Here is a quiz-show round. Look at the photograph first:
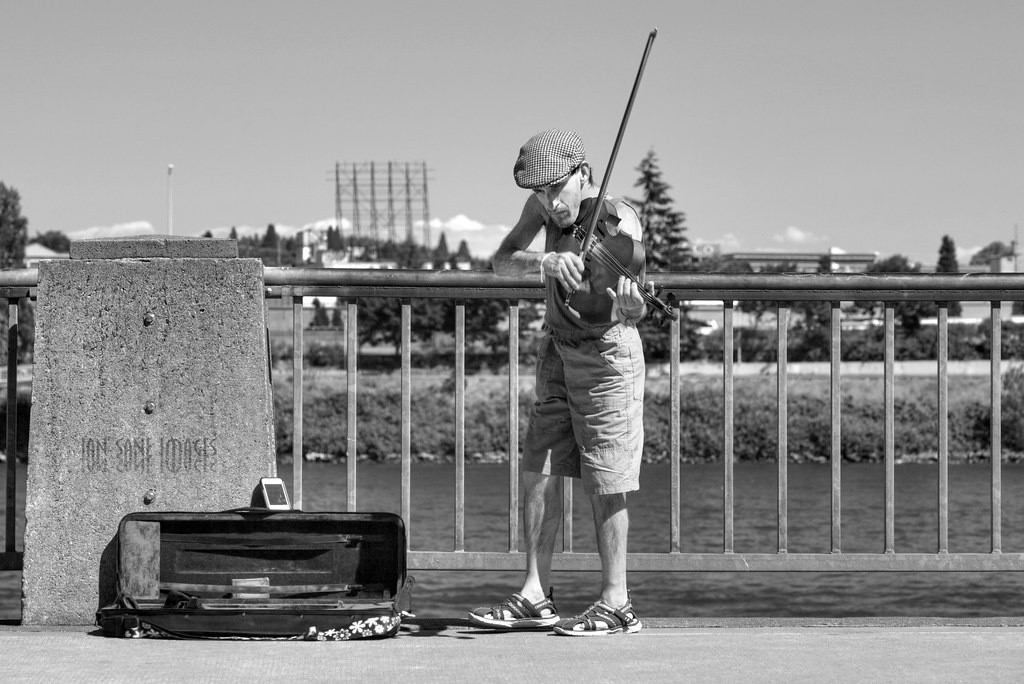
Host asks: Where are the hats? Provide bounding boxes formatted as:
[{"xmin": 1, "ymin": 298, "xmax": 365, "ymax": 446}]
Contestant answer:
[{"xmin": 513, "ymin": 129, "xmax": 585, "ymax": 187}]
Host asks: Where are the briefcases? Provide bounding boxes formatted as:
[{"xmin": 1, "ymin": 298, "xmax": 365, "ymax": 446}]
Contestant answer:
[{"xmin": 95, "ymin": 507, "xmax": 414, "ymax": 641}]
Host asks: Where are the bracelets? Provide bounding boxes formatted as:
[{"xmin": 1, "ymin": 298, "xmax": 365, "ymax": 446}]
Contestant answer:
[
  {"xmin": 620, "ymin": 308, "xmax": 642, "ymax": 329},
  {"xmin": 540, "ymin": 252, "xmax": 556, "ymax": 283}
]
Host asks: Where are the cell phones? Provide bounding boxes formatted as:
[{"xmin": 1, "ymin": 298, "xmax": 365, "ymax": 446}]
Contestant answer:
[{"xmin": 260, "ymin": 477, "xmax": 290, "ymax": 510}]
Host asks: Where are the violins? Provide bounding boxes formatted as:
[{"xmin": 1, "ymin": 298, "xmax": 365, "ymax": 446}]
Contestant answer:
[{"xmin": 554, "ymin": 195, "xmax": 679, "ymax": 328}]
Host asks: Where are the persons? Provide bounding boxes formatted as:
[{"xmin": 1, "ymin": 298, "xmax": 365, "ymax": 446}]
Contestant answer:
[{"xmin": 468, "ymin": 130, "xmax": 654, "ymax": 637}]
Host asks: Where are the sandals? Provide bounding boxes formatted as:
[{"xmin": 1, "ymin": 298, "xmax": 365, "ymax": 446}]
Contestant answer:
[
  {"xmin": 553, "ymin": 588, "xmax": 643, "ymax": 636},
  {"xmin": 467, "ymin": 586, "xmax": 560, "ymax": 628}
]
